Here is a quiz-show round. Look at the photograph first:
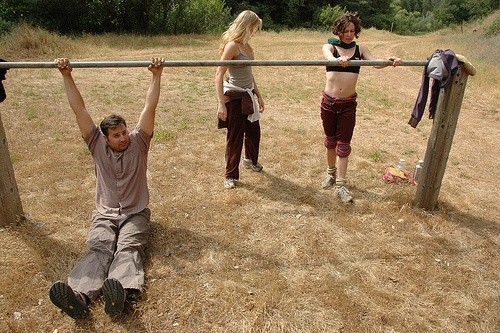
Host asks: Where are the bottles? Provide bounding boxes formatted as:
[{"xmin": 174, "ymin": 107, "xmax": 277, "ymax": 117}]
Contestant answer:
[
  {"xmin": 413, "ymin": 160, "xmax": 424, "ymax": 182},
  {"xmin": 396, "ymin": 159, "xmax": 405, "ymax": 172}
]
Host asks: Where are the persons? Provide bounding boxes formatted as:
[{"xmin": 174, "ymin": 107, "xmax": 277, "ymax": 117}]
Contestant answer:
[
  {"xmin": 216, "ymin": 10, "xmax": 265, "ymax": 189},
  {"xmin": 320, "ymin": 14, "xmax": 401, "ymax": 203},
  {"xmin": 49, "ymin": 57, "xmax": 165, "ymax": 320}
]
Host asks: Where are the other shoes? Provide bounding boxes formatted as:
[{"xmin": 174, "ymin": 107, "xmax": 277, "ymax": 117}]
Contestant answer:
[
  {"xmin": 223, "ymin": 178, "xmax": 235, "ymax": 189},
  {"xmin": 321, "ymin": 175, "xmax": 335, "ymax": 189},
  {"xmin": 332, "ymin": 186, "xmax": 353, "ymax": 202},
  {"xmin": 101, "ymin": 277, "xmax": 126, "ymax": 317},
  {"xmin": 49, "ymin": 281, "xmax": 89, "ymax": 319},
  {"xmin": 242, "ymin": 156, "xmax": 263, "ymax": 172}
]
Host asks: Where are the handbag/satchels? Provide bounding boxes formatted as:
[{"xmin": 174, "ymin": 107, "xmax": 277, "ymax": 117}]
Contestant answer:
[{"xmin": 383, "ymin": 165, "xmax": 418, "ymax": 186}]
